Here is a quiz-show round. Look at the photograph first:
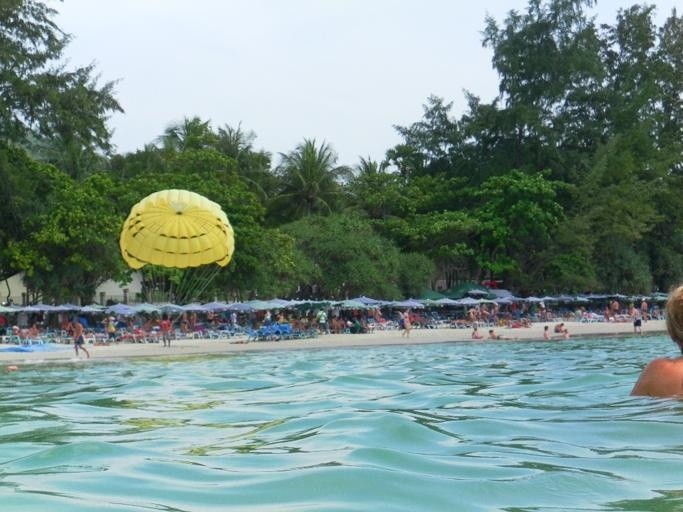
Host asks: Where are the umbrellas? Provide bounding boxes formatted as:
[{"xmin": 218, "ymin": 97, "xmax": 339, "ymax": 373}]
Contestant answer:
[{"xmin": 524, "ymin": 292, "xmax": 667, "ymax": 301}]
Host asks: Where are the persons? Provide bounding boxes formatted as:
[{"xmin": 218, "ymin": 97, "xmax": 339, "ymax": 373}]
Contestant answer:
[
  {"xmin": 1, "ymin": 297, "xmax": 663, "ymax": 338},
  {"xmin": 544, "ymin": 326, "xmax": 550, "ymax": 340},
  {"xmin": 65, "ymin": 318, "xmax": 90, "ymax": 359},
  {"xmin": 161, "ymin": 312, "xmax": 172, "ymax": 348},
  {"xmin": 628, "ymin": 286, "xmax": 683, "ymax": 400}
]
